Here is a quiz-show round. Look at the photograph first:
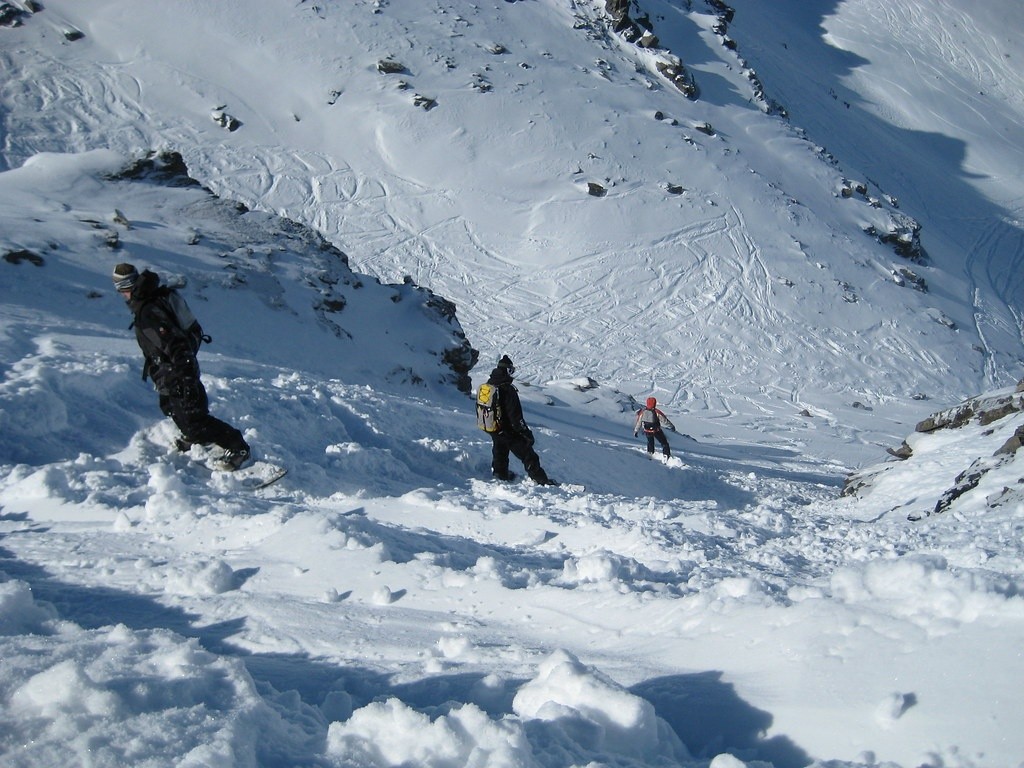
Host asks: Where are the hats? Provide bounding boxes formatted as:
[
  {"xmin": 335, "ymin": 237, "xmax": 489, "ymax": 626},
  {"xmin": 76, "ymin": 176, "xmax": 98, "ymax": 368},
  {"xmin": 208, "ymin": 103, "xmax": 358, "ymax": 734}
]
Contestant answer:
[
  {"xmin": 112, "ymin": 263, "xmax": 140, "ymax": 291},
  {"xmin": 498, "ymin": 355, "xmax": 515, "ymax": 370},
  {"xmin": 647, "ymin": 397, "xmax": 656, "ymax": 409}
]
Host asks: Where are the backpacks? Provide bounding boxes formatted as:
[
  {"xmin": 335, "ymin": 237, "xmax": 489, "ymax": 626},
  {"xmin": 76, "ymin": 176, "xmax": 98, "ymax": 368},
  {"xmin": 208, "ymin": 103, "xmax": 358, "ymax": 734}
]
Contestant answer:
[
  {"xmin": 475, "ymin": 381, "xmax": 511, "ymax": 434},
  {"xmin": 641, "ymin": 407, "xmax": 660, "ymax": 430},
  {"xmin": 134, "ymin": 284, "xmax": 212, "ymax": 360}
]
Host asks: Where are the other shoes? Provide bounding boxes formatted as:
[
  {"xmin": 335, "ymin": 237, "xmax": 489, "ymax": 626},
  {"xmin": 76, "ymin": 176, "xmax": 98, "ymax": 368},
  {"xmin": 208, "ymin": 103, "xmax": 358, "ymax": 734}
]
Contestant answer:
[
  {"xmin": 173, "ymin": 434, "xmax": 214, "ymax": 452},
  {"xmin": 493, "ymin": 470, "xmax": 515, "ymax": 481},
  {"xmin": 537, "ymin": 479, "xmax": 558, "ymax": 487},
  {"xmin": 214, "ymin": 446, "xmax": 251, "ymax": 472}
]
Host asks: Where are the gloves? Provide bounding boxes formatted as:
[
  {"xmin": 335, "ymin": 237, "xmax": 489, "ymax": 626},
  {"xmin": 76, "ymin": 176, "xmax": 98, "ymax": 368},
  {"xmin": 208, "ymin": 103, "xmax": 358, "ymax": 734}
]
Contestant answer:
[
  {"xmin": 634, "ymin": 431, "xmax": 638, "ymax": 438},
  {"xmin": 520, "ymin": 426, "xmax": 535, "ymax": 446},
  {"xmin": 669, "ymin": 427, "xmax": 676, "ymax": 433}
]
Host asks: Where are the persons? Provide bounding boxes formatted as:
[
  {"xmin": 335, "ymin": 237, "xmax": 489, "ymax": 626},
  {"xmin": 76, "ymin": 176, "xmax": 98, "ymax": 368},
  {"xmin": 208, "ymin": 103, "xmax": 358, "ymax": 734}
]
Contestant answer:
[
  {"xmin": 634, "ymin": 397, "xmax": 675, "ymax": 459},
  {"xmin": 113, "ymin": 263, "xmax": 251, "ymax": 472},
  {"xmin": 486, "ymin": 355, "xmax": 557, "ymax": 486}
]
